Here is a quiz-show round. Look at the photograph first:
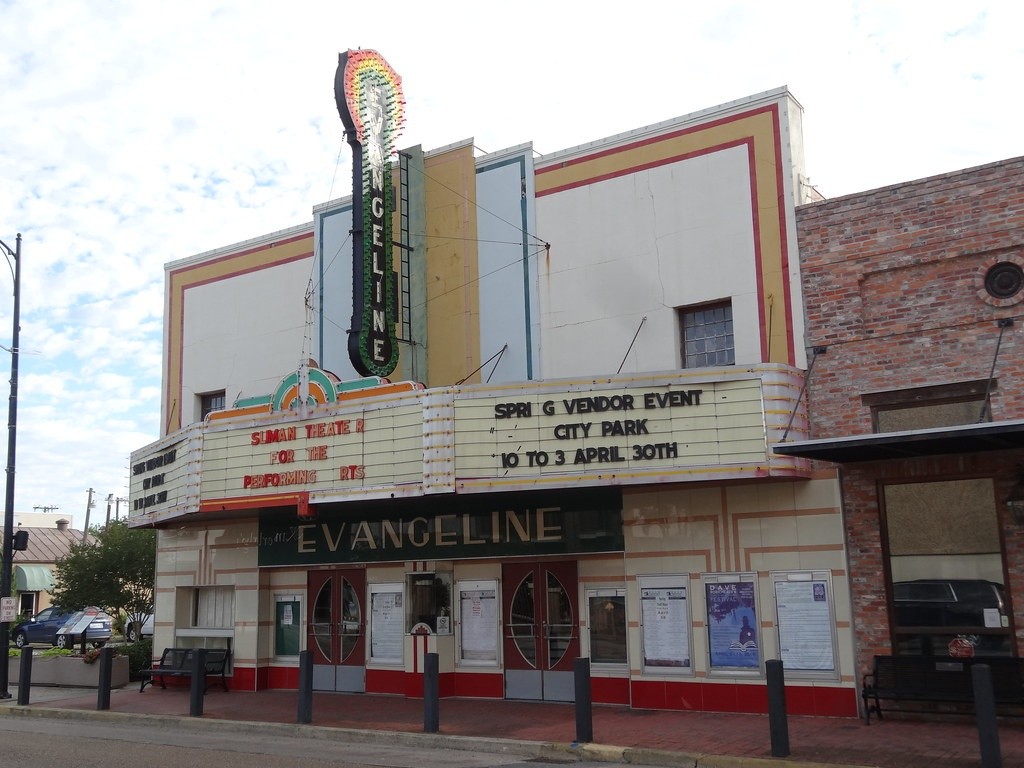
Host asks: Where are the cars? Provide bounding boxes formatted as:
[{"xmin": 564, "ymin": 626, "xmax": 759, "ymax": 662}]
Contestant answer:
[
  {"xmin": 892, "ymin": 577, "xmax": 1005, "ymax": 629},
  {"xmin": 11, "ymin": 606, "xmax": 113, "ymax": 650},
  {"xmin": 125, "ymin": 602, "xmax": 154, "ymax": 644}
]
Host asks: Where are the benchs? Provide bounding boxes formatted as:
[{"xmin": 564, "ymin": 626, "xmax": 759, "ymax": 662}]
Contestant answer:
[
  {"xmin": 139, "ymin": 648, "xmax": 231, "ymax": 695},
  {"xmin": 862, "ymin": 655, "xmax": 1024, "ymax": 725}
]
